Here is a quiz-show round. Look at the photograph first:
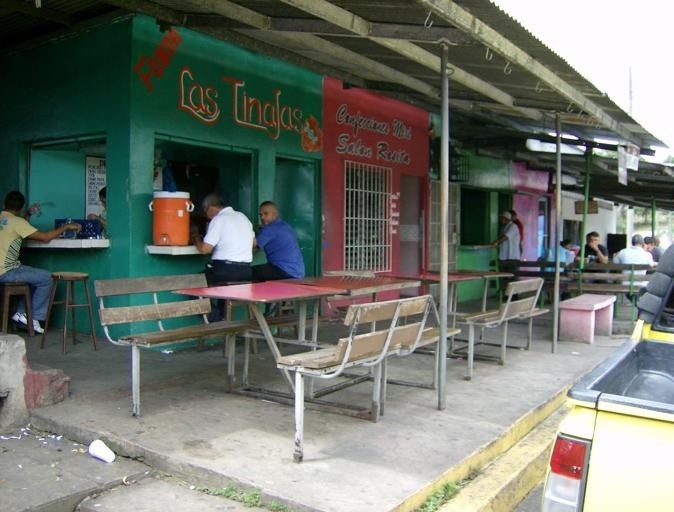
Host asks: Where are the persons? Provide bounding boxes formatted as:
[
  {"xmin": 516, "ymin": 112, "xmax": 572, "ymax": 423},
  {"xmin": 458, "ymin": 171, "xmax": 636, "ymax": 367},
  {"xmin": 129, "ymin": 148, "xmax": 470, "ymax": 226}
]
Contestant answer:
[
  {"xmin": 551, "ymin": 233, "xmax": 663, "ymax": 302},
  {"xmin": 491, "ymin": 211, "xmax": 520, "ymax": 302},
  {"xmin": 0, "ymin": 191, "xmax": 82, "ymax": 335},
  {"xmin": 86, "ymin": 185, "xmax": 106, "ymax": 236},
  {"xmin": 256, "ymin": 201, "xmax": 305, "ymax": 318},
  {"xmin": 510, "ymin": 210, "xmax": 523, "ymax": 257},
  {"xmin": 189, "ymin": 192, "xmax": 258, "ymax": 324}
]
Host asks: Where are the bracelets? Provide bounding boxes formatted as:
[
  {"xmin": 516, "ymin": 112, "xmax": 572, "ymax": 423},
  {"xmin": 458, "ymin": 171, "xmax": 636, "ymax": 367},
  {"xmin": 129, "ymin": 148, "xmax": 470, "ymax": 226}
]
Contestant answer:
[
  {"xmin": 27, "ymin": 211, "xmax": 32, "ymax": 216},
  {"xmin": 493, "ymin": 241, "xmax": 498, "ymax": 246}
]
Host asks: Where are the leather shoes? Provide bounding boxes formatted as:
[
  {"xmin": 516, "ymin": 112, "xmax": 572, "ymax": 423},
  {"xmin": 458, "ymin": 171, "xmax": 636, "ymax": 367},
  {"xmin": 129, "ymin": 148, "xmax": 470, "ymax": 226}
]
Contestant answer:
[{"xmin": 263, "ymin": 303, "xmax": 276, "ymax": 318}]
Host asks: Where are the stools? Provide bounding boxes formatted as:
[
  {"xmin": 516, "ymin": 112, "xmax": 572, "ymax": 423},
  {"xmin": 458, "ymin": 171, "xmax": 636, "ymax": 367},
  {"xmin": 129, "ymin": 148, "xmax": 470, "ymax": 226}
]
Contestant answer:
[
  {"xmin": 42, "ymin": 272, "xmax": 99, "ymax": 355},
  {"xmin": 0, "ymin": 280, "xmax": 35, "ymax": 336}
]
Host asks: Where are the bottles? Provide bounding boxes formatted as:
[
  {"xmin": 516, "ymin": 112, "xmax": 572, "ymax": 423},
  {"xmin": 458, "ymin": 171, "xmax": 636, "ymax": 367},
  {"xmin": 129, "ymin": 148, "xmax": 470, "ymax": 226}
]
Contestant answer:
[{"xmin": 63, "ymin": 217, "xmax": 75, "ymax": 239}]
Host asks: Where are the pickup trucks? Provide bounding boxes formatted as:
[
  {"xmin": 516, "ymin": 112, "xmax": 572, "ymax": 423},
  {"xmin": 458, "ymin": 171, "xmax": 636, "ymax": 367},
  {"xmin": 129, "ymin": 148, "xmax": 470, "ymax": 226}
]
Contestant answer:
[{"xmin": 541, "ymin": 243, "xmax": 673, "ymax": 512}]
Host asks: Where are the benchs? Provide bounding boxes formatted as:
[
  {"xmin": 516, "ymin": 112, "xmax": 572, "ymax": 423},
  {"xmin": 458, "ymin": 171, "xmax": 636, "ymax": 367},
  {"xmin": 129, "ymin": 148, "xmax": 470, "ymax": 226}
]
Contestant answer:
[
  {"xmin": 489, "ymin": 258, "xmax": 563, "ymax": 319},
  {"xmin": 565, "ymin": 262, "xmax": 651, "ymax": 325},
  {"xmin": 557, "ymin": 292, "xmax": 617, "ymax": 344}
]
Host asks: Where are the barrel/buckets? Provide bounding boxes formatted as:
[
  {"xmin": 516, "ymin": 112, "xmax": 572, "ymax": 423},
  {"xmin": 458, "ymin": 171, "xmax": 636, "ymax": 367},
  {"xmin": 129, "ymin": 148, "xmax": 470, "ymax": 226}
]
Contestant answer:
[{"xmin": 148, "ymin": 189, "xmax": 194, "ymax": 246}]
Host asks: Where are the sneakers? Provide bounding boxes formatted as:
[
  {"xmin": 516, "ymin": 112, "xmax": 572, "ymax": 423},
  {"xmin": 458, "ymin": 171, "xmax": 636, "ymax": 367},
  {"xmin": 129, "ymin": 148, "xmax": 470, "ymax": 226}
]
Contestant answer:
[{"xmin": 12, "ymin": 312, "xmax": 44, "ymax": 334}]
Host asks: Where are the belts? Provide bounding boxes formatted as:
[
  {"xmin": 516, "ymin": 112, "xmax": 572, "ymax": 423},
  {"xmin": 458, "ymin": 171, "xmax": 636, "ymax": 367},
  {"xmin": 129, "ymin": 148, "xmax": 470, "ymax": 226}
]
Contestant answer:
[{"xmin": 224, "ymin": 261, "xmax": 249, "ymax": 266}]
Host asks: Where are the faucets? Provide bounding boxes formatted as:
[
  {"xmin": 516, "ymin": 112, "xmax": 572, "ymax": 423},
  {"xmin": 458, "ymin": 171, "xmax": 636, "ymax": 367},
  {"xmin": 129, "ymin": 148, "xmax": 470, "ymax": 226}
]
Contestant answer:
[{"xmin": 160, "ymin": 232, "xmax": 169, "ymax": 242}]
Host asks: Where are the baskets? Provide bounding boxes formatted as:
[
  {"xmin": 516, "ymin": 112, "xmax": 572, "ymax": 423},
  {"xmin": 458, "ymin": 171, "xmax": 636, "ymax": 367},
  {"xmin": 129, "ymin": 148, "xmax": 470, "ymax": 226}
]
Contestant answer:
[{"xmin": 55, "ymin": 219, "xmax": 101, "ymax": 239}]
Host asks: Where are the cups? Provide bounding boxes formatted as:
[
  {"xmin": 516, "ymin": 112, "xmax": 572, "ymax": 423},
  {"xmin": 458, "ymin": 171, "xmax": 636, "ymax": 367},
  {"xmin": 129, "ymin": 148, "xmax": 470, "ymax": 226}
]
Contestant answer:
[{"xmin": 30, "ymin": 205, "xmax": 41, "ymax": 218}]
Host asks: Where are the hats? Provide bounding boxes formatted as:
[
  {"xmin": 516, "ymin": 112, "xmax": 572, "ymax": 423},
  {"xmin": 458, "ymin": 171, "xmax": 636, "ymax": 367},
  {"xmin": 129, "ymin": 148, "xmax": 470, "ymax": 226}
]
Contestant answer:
[{"xmin": 499, "ymin": 211, "xmax": 511, "ymax": 219}]
